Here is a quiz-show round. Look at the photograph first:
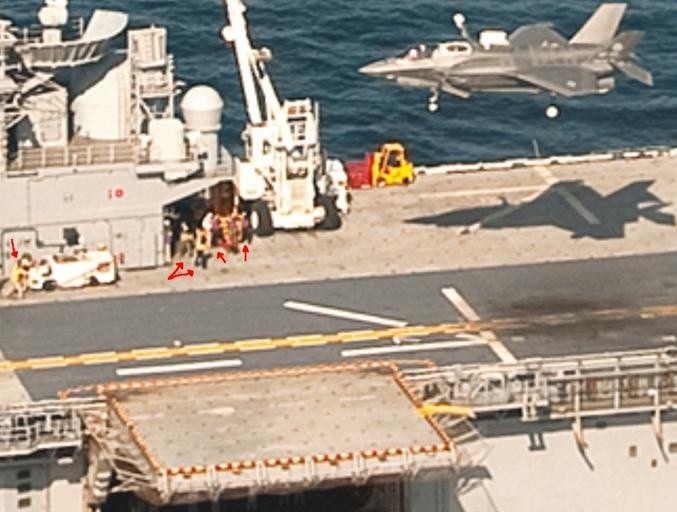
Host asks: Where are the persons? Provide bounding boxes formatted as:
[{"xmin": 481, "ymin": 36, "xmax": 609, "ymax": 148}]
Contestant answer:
[
  {"xmin": 174, "ymin": 207, "xmax": 254, "ymax": 269},
  {"xmin": 7, "ymin": 258, "xmax": 31, "ymax": 299}
]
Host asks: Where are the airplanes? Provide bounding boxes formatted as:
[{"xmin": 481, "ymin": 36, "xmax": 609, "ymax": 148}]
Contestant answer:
[{"xmin": 352, "ymin": 1, "xmax": 657, "ymax": 121}]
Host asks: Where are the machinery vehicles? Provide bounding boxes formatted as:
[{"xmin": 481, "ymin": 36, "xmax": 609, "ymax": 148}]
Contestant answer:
[
  {"xmin": 27, "ymin": 248, "xmax": 119, "ymax": 294},
  {"xmin": 215, "ymin": 1, "xmax": 349, "ymax": 239}
]
